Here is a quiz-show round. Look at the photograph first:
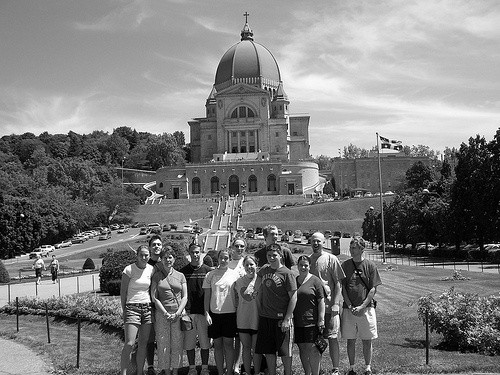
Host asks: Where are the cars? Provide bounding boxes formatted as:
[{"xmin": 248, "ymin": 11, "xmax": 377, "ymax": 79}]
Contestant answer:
[
  {"xmin": 259, "ymin": 191, "xmax": 394, "ymax": 212},
  {"xmin": 181, "ymin": 225, "xmax": 203, "ymax": 233},
  {"xmin": 385, "ymin": 242, "xmax": 500, "ymax": 253},
  {"xmin": 29, "ymin": 223, "xmax": 129, "ymax": 260},
  {"xmin": 139, "ymin": 223, "xmax": 178, "ymax": 236},
  {"xmin": 236, "ymin": 225, "xmax": 350, "ymax": 243},
  {"xmin": 131, "ymin": 221, "xmax": 146, "ymax": 229},
  {"xmin": 423, "ymin": 189, "xmax": 429, "ymax": 194}
]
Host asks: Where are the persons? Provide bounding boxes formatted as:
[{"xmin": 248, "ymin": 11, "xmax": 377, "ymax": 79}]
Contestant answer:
[
  {"xmin": 294, "ymin": 256, "xmax": 326, "ymax": 375},
  {"xmin": 340, "ymin": 236, "xmax": 383, "ymax": 375},
  {"xmin": 178, "ymin": 243, "xmax": 215, "ymax": 375},
  {"xmin": 46, "ymin": 255, "xmax": 59, "ymax": 282},
  {"xmin": 254, "ymin": 224, "xmax": 296, "ymax": 375},
  {"xmin": 307, "ymin": 231, "xmax": 346, "ymax": 375},
  {"xmin": 32, "ymin": 252, "xmax": 47, "ymax": 283},
  {"xmin": 130, "ymin": 236, "xmax": 162, "ymax": 375},
  {"xmin": 222, "ymin": 238, "xmax": 251, "ymax": 375},
  {"xmin": 234, "ymin": 255, "xmax": 263, "ymax": 375},
  {"xmin": 254, "ymin": 244, "xmax": 297, "ymax": 374},
  {"xmin": 120, "ymin": 245, "xmax": 155, "ymax": 375},
  {"xmin": 151, "ymin": 246, "xmax": 188, "ymax": 375},
  {"xmin": 202, "ymin": 248, "xmax": 241, "ymax": 375}
]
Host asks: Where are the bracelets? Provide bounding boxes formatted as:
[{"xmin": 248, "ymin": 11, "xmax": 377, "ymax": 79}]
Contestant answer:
[
  {"xmin": 175, "ymin": 313, "xmax": 178, "ymax": 320},
  {"xmin": 204, "ymin": 312, "xmax": 209, "ymax": 316}
]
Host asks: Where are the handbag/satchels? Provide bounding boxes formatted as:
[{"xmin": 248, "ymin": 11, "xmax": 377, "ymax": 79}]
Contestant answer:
[
  {"xmin": 180, "ymin": 313, "xmax": 193, "ymax": 331},
  {"xmin": 314, "ymin": 326, "xmax": 328, "ymax": 355}
]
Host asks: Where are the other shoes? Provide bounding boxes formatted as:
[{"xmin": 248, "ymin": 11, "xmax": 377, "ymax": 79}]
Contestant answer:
[
  {"xmin": 188, "ymin": 368, "xmax": 197, "ymax": 375},
  {"xmin": 319, "ymin": 370, "xmax": 325, "ymax": 375},
  {"xmin": 331, "ymin": 369, "xmax": 339, "ymax": 375},
  {"xmin": 200, "ymin": 368, "xmax": 210, "ymax": 375},
  {"xmin": 148, "ymin": 368, "xmax": 156, "ymax": 375},
  {"xmin": 348, "ymin": 368, "xmax": 357, "ymax": 375},
  {"xmin": 364, "ymin": 370, "xmax": 373, "ymax": 375}
]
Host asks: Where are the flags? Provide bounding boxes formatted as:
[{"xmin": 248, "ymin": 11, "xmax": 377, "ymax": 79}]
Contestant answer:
[{"xmin": 377, "ymin": 135, "xmax": 404, "ymax": 155}]
